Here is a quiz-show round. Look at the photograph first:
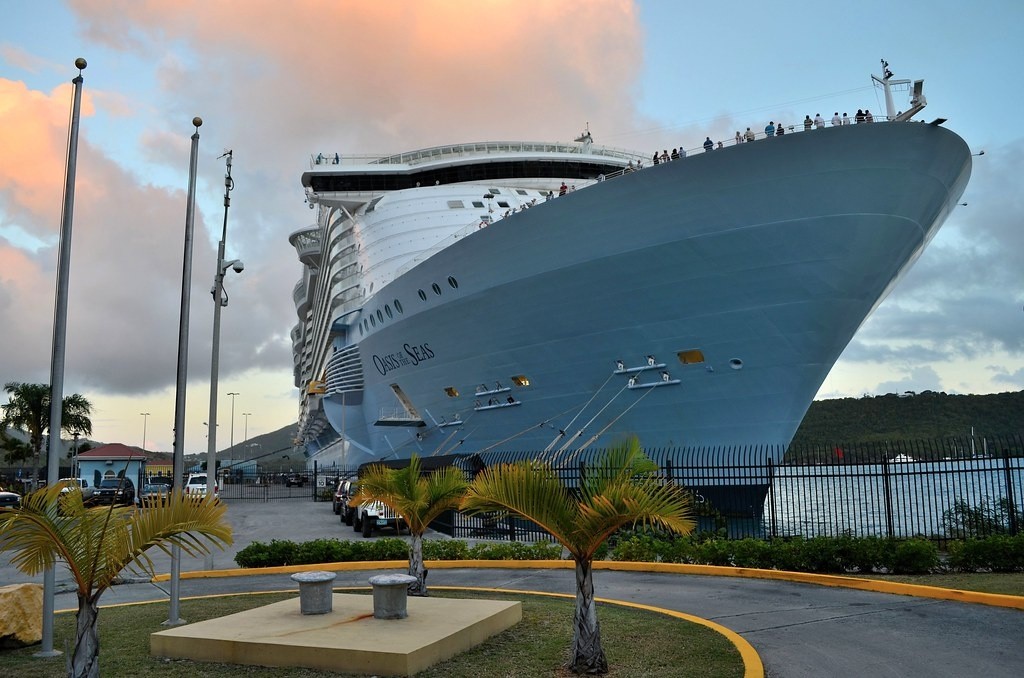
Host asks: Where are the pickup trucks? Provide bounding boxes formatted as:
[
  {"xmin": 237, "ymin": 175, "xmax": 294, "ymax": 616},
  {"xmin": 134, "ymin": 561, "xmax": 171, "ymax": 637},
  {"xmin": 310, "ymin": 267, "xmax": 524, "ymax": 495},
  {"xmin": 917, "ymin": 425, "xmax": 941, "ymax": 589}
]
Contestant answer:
[{"xmin": 58, "ymin": 478, "xmax": 96, "ymax": 501}]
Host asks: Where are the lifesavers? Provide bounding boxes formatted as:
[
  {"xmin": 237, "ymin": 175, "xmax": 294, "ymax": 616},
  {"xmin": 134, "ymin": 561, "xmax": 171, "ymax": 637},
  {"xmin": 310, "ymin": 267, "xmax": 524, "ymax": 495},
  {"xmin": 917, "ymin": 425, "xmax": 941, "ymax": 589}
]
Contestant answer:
[{"xmin": 479, "ymin": 222, "xmax": 488, "ymax": 229}]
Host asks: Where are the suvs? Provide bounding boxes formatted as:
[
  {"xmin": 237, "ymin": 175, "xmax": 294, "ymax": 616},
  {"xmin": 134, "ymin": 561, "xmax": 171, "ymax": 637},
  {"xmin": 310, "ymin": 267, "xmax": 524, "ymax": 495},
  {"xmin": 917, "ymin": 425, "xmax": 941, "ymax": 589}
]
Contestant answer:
[
  {"xmin": 183, "ymin": 472, "xmax": 219, "ymax": 505},
  {"xmin": 92, "ymin": 477, "xmax": 135, "ymax": 504}
]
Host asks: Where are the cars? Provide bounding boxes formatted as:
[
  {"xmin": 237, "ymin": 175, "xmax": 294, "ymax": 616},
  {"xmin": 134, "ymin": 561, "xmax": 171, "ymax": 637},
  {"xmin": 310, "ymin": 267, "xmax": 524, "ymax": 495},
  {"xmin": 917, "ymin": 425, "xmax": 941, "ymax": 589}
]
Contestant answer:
[
  {"xmin": 332, "ymin": 479, "xmax": 423, "ymax": 538},
  {"xmin": 140, "ymin": 482, "xmax": 171, "ymax": 506},
  {"xmin": 288, "ymin": 473, "xmax": 303, "ymax": 486},
  {"xmin": 0, "ymin": 491, "xmax": 23, "ymax": 514}
]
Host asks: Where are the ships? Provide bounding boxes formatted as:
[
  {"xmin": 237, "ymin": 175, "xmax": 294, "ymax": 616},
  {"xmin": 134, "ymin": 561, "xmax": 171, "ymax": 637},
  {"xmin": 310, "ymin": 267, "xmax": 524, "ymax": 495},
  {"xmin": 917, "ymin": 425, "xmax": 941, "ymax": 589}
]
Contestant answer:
[{"xmin": 289, "ymin": 56, "xmax": 973, "ymax": 541}]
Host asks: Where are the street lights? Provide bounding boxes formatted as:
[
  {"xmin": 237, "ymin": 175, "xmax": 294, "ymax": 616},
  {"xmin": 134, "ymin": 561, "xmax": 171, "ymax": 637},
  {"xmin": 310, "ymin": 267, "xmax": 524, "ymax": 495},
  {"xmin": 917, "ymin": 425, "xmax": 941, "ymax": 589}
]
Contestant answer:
[
  {"xmin": 139, "ymin": 412, "xmax": 150, "ymax": 453},
  {"xmin": 228, "ymin": 392, "xmax": 241, "ymax": 467},
  {"xmin": 242, "ymin": 412, "xmax": 251, "ymax": 443}
]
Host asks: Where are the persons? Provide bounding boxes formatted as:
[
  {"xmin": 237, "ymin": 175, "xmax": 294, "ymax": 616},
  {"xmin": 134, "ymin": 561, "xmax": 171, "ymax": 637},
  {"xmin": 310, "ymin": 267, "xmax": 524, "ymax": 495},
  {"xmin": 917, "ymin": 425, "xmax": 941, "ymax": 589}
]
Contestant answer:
[
  {"xmin": 897, "ymin": 111, "xmax": 902, "ymax": 116},
  {"xmin": 765, "ymin": 121, "xmax": 775, "ymax": 137},
  {"xmin": 832, "ymin": 112, "xmax": 850, "ymax": 126},
  {"xmin": 16, "ymin": 469, "xmax": 23, "ymax": 479},
  {"xmin": 505, "ymin": 199, "xmax": 537, "ymax": 217},
  {"xmin": 804, "ymin": 115, "xmax": 813, "ymax": 130},
  {"xmin": 703, "ymin": 137, "xmax": 723, "ymax": 151},
  {"xmin": 334, "ymin": 153, "xmax": 340, "ymax": 164},
  {"xmin": 627, "ymin": 160, "xmax": 643, "ymax": 172},
  {"xmin": 736, "ymin": 127, "xmax": 755, "ymax": 144},
  {"xmin": 855, "ymin": 109, "xmax": 872, "ymax": 123},
  {"xmin": 814, "ymin": 114, "xmax": 825, "ymax": 129},
  {"xmin": 546, "ymin": 191, "xmax": 554, "ymax": 199},
  {"xmin": 653, "ymin": 147, "xmax": 686, "ymax": 166},
  {"xmin": 559, "ymin": 182, "xmax": 567, "ymax": 196},
  {"xmin": 316, "ymin": 153, "xmax": 325, "ymax": 164},
  {"xmin": 776, "ymin": 124, "xmax": 784, "ymax": 136},
  {"xmin": 885, "ymin": 69, "xmax": 891, "ymax": 76}
]
